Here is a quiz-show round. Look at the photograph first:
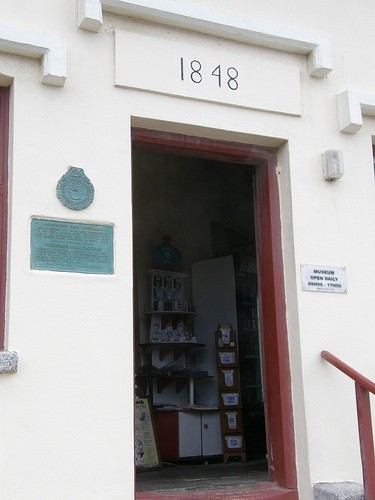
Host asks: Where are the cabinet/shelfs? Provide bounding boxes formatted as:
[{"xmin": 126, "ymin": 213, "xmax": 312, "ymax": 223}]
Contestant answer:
[
  {"xmin": 233, "ymin": 269, "xmax": 264, "ymax": 428},
  {"xmin": 132, "ymin": 230, "xmax": 245, "ymax": 464}
]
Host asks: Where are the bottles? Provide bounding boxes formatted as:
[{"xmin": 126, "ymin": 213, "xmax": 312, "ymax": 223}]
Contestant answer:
[
  {"xmin": 164, "ymin": 272, "xmax": 172, "ymax": 297},
  {"xmin": 153, "ymin": 271, "xmax": 163, "ymax": 299},
  {"xmin": 174, "ymin": 274, "xmax": 181, "ymax": 290}
]
